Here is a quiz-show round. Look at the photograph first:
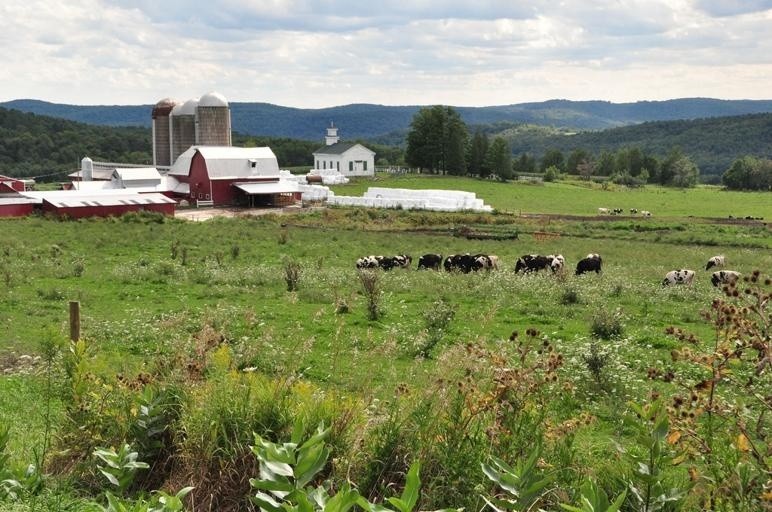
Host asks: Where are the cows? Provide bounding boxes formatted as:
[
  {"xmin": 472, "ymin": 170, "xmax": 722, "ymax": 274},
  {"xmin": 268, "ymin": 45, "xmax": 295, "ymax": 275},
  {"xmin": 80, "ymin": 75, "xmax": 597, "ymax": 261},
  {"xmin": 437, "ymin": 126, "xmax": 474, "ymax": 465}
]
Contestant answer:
[
  {"xmin": 661, "ymin": 269, "xmax": 696, "ymax": 288},
  {"xmin": 702, "ymin": 256, "xmax": 727, "ymax": 271},
  {"xmin": 598, "ymin": 207, "xmax": 653, "ymax": 217},
  {"xmin": 587, "ymin": 253, "xmax": 601, "ymax": 262},
  {"xmin": 711, "ymin": 270, "xmax": 742, "ymax": 289},
  {"xmin": 575, "ymin": 259, "xmax": 600, "ymax": 276},
  {"xmin": 514, "ymin": 253, "xmax": 565, "ymax": 276},
  {"xmin": 355, "ymin": 252, "xmax": 500, "ymax": 275}
]
